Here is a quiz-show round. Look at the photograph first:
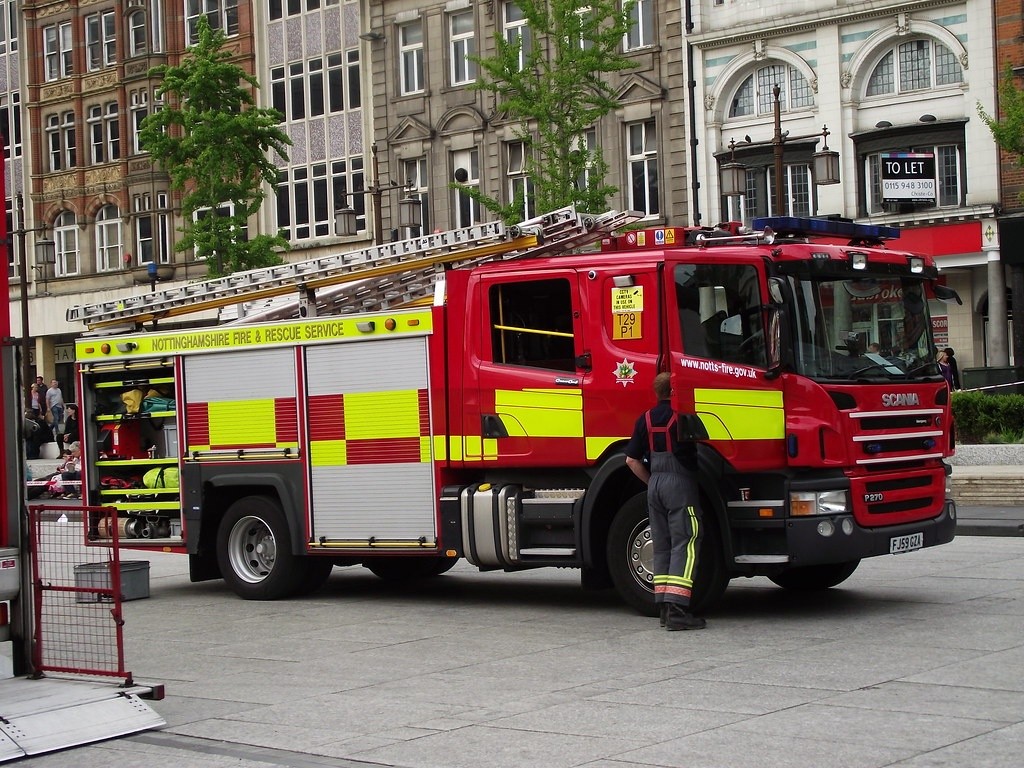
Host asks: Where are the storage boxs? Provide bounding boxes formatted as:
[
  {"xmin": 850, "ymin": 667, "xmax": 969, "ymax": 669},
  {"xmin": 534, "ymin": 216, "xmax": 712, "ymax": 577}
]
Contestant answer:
[{"xmin": 74, "ymin": 561, "xmax": 150, "ymax": 603}]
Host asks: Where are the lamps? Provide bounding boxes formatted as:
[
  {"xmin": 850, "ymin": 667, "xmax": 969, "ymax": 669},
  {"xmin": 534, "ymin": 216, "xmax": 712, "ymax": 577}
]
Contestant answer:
[{"xmin": 359, "ymin": 33, "xmax": 384, "ymax": 42}]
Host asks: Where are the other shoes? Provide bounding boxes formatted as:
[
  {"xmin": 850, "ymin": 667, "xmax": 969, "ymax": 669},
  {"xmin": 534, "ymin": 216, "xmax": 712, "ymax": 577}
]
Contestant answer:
[
  {"xmin": 660, "ymin": 605, "xmax": 666, "ymax": 627},
  {"xmin": 77, "ymin": 494, "xmax": 83, "ymax": 500},
  {"xmin": 63, "ymin": 492, "xmax": 78, "ymax": 500},
  {"xmin": 665, "ymin": 602, "xmax": 707, "ymax": 630}
]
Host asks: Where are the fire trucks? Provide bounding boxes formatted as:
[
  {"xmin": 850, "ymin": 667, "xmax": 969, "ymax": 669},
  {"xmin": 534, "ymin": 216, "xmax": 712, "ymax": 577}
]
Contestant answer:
[{"xmin": 65, "ymin": 199, "xmax": 959, "ymax": 610}]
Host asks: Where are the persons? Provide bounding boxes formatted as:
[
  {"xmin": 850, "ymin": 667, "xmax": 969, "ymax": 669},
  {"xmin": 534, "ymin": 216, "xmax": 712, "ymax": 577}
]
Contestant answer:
[
  {"xmin": 30, "ymin": 376, "xmax": 48, "ymax": 422},
  {"xmin": 937, "ymin": 348, "xmax": 961, "ymax": 392},
  {"xmin": 46, "ymin": 379, "xmax": 65, "ymax": 435},
  {"xmin": 625, "ymin": 373, "xmax": 709, "ymax": 631},
  {"xmin": 56, "ymin": 404, "xmax": 79, "ymax": 459},
  {"xmin": 27, "ymin": 441, "xmax": 83, "ymax": 500},
  {"xmin": 25, "ymin": 408, "xmax": 55, "ymax": 459},
  {"xmin": 868, "ymin": 343, "xmax": 880, "ymax": 353},
  {"xmin": 893, "ymin": 345, "xmax": 904, "ymax": 356}
]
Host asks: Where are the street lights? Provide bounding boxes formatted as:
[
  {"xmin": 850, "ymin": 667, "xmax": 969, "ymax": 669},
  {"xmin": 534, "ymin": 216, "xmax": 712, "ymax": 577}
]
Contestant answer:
[
  {"xmin": 328, "ymin": 139, "xmax": 424, "ymax": 245},
  {"xmin": 712, "ymin": 83, "xmax": 844, "ymax": 224},
  {"xmin": 6, "ymin": 192, "xmax": 58, "ymax": 454}
]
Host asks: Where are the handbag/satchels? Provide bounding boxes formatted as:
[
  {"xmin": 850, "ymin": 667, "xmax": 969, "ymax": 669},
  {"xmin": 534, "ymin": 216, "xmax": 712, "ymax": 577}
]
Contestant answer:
[{"xmin": 44, "ymin": 410, "xmax": 55, "ymax": 424}]
